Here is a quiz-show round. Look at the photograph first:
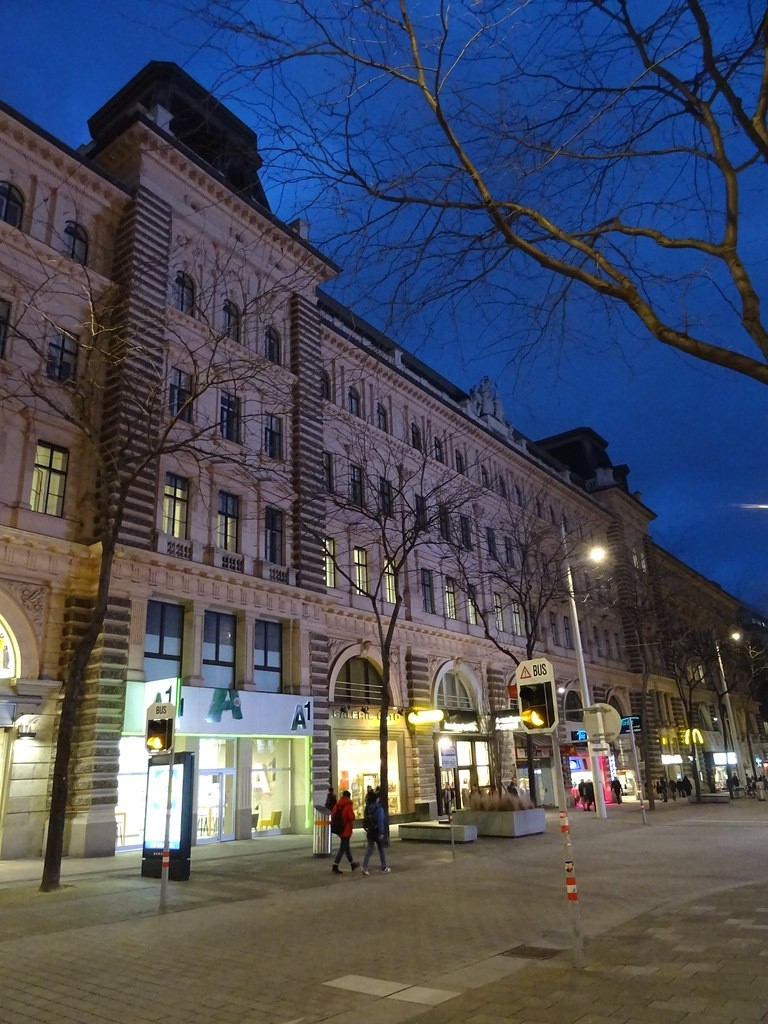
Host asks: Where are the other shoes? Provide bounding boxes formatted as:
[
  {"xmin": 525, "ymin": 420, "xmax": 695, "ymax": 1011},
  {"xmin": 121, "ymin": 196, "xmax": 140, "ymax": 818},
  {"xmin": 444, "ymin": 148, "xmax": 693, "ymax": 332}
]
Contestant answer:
[
  {"xmin": 363, "ymin": 870, "xmax": 369, "ymax": 875},
  {"xmin": 351, "ymin": 862, "xmax": 359, "ymax": 871},
  {"xmin": 588, "ymin": 809, "xmax": 591, "ymax": 811},
  {"xmin": 584, "ymin": 809, "xmax": 586, "ymax": 811},
  {"xmin": 382, "ymin": 866, "xmax": 391, "ymax": 873},
  {"xmin": 663, "ymin": 800, "xmax": 667, "ymax": 802},
  {"xmin": 332, "ymin": 864, "xmax": 343, "ymax": 874}
]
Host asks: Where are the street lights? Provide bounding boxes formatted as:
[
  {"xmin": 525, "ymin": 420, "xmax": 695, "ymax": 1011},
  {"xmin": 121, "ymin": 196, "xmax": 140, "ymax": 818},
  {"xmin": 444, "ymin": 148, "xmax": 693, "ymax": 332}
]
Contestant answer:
[
  {"xmin": 711, "ymin": 626, "xmax": 749, "ymax": 800},
  {"xmin": 560, "ymin": 521, "xmax": 609, "ymax": 823}
]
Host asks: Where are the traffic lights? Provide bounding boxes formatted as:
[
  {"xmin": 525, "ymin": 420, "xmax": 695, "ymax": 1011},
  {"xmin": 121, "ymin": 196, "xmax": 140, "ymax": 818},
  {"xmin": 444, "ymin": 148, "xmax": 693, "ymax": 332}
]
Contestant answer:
[
  {"xmin": 148, "ymin": 719, "xmax": 166, "ymax": 750},
  {"xmin": 513, "ymin": 657, "xmax": 560, "ymax": 736}
]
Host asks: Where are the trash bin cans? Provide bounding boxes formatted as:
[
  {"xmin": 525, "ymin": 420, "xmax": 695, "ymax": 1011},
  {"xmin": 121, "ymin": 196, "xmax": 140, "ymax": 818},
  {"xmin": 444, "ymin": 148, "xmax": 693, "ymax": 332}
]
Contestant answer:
[
  {"xmin": 313, "ymin": 804, "xmax": 331, "ymax": 856},
  {"xmin": 756, "ymin": 782, "xmax": 766, "ymax": 800}
]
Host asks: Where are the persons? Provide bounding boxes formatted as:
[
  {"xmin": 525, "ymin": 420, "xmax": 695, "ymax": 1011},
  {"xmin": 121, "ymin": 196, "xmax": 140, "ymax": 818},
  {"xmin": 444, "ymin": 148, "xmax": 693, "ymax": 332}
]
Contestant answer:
[
  {"xmin": 375, "ymin": 786, "xmax": 382, "ymax": 807},
  {"xmin": 586, "ymin": 779, "xmax": 596, "ymax": 812},
  {"xmin": 611, "ymin": 777, "xmax": 622, "ymax": 804},
  {"xmin": 732, "ymin": 772, "xmax": 768, "ymax": 795},
  {"xmin": 332, "ymin": 790, "xmax": 360, "ymax": 874},
  {"xmin": 325, "ymin": 787, "xmax": 337, "ymax": 811},
  {"xmin": 578, "ymin": 779, "xmax": 589, "ymax": 811},
  {"xmin": 360, "ymin": 793, "xmax": 391, "ymax": 875},
  {"xmin": 441, "ymin": 782, "xmax": 454, "ymax": 815},
  {"xmin": 645, "ymin": 775, "xmax": 693, "ymax": 802},
  {"xmin": 365, "ymin": 785, "xmax": 376, "ymax": 828}
]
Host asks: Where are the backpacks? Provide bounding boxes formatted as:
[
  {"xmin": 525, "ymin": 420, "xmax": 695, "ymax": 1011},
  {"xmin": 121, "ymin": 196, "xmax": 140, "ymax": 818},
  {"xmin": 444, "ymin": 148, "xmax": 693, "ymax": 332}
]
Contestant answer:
[
  {"xmin": 331, "ymin": 804, "xmax": 344, "ymax": 835},
  {"xmin": 362, "ymin": 806, "xmax": 381, "ymax": 833}
]
